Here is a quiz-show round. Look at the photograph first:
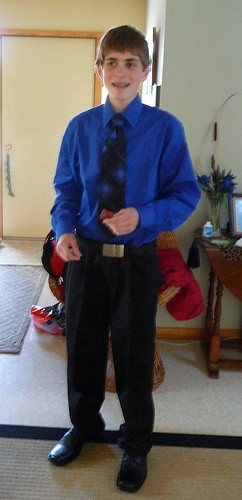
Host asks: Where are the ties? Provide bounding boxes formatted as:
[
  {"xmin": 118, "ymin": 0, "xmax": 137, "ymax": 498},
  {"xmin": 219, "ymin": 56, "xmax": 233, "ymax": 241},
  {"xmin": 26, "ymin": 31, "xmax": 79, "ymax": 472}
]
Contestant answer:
[{"xmin": 98, "ymin": 115, "xmax": 126, "ymax": 239}]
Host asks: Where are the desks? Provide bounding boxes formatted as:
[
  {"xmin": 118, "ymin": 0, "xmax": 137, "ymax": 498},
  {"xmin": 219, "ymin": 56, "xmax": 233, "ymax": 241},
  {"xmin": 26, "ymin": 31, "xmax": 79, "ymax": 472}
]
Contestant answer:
[{"xmin": 193, "ymin": 229, "xmax": 242, "ymax": 380}]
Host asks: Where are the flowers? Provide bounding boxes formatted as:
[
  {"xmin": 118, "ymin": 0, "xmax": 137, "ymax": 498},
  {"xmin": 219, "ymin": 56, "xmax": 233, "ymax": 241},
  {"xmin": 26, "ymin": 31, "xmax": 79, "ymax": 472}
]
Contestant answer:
[{"xmin": 195, "ymin": 165, "xmax": 238, "ymax": 228}]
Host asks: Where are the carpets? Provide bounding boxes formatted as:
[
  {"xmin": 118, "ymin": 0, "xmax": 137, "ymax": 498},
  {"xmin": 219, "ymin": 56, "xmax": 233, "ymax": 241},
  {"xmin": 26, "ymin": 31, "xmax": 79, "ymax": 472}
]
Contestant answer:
[
  {"xmin": 0, "ymin": 264, "xmax": 49, "ymax": 355},
  {"xmin": 0, "ymin": 424, "xmax": 242, "ymax": 500}
]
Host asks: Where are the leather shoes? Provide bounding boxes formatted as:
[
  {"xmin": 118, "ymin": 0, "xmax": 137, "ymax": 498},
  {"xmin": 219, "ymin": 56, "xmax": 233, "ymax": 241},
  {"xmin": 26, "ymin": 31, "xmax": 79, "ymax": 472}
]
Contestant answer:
[
  {"xmin": 47, "ymin": 421, "xmax": 105, "ymax": 466},
  {"xmin": 115, "ymin": 450, "xmax": 147, "ymax": 494}
]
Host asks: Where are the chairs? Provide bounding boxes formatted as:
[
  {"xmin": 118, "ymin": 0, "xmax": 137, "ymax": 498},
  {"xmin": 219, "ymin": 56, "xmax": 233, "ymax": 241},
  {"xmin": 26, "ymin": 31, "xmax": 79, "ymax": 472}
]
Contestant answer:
[{"xmin": 41, "ymin": 226, "xmax": 185, "ymax": 393}]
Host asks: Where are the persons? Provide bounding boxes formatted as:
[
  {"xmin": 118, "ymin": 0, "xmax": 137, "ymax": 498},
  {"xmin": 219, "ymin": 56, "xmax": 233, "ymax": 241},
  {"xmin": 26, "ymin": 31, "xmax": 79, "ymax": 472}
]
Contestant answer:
[{"xmin": 49, "ymin": 25, "xmax": 201, "ymax": 492}]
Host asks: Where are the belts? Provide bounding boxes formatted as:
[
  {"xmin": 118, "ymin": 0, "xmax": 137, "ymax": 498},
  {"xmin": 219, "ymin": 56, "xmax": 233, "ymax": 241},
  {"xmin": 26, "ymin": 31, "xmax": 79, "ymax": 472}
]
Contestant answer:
[{"xmin": 77, "ymin": 235, "xmax": 157, "ymax": 258}]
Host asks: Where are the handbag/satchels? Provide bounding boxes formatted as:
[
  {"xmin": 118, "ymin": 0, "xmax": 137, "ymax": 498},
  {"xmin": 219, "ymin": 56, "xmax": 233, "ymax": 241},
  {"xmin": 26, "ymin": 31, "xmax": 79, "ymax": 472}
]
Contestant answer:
[{"xmin": 30, "ymin": 300, "xmax": 67, "ymax": 336}]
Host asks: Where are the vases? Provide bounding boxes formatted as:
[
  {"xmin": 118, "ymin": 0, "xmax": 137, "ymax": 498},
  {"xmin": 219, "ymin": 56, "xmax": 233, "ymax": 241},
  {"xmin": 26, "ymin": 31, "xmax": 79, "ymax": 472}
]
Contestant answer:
[{"xmin": 209, "ymin": 197, "xmax": 223, "ymax": 240}]
